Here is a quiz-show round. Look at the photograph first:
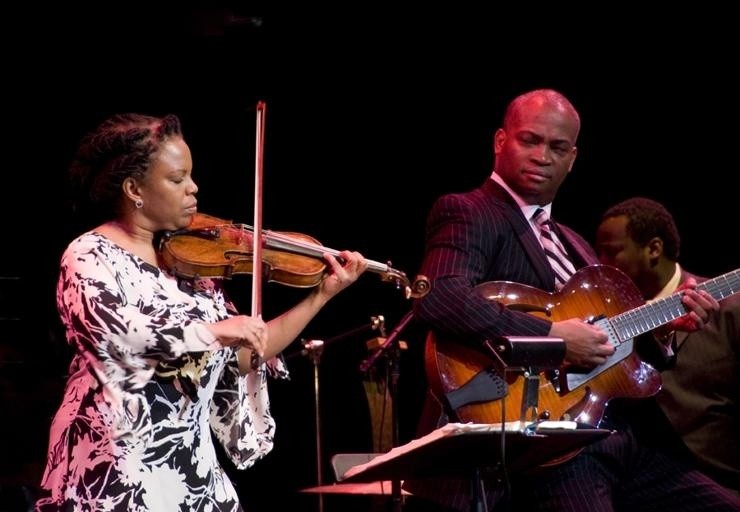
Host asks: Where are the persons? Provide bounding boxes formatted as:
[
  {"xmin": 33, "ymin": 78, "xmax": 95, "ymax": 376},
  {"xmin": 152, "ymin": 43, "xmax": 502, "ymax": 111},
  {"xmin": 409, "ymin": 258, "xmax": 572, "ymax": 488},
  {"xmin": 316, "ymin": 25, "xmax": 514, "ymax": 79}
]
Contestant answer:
[
  {"xmin": 596, "ymin": 197, "xmax": 738, "ymax": 491},
  {"xmin": 412, "ymin": 89, "xmax": 739, "ymax": 511},
  {"xmin": 31, "ymin": 111, "xmax": 366, "ymax": 512}
]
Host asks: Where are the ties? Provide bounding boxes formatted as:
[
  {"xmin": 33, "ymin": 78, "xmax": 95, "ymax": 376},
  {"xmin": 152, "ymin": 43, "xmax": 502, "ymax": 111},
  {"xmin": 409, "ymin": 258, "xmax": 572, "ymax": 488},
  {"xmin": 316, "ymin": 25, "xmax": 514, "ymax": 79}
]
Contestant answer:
[{"xmin": 531, "ymin": 208, "xmax": 578, "ymax": 295}]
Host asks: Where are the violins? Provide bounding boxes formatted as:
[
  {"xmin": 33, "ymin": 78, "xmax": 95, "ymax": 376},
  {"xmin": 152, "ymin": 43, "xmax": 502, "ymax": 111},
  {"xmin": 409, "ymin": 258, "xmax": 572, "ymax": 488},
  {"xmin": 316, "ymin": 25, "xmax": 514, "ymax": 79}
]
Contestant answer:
[{"xmin": 154, "ymin": 212, "xmax": 431, "ymax": 301}]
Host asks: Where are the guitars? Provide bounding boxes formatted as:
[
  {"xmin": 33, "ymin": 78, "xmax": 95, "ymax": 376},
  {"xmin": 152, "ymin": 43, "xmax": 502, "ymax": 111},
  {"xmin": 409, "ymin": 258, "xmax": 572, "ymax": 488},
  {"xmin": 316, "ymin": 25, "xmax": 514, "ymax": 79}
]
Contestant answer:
[{"xmin": 428, "ymin": 261, "xmax": 739, "ymax": 472}]
